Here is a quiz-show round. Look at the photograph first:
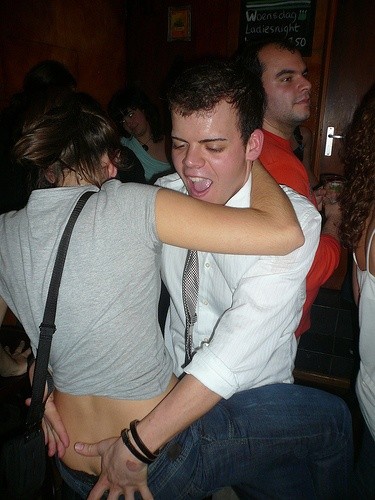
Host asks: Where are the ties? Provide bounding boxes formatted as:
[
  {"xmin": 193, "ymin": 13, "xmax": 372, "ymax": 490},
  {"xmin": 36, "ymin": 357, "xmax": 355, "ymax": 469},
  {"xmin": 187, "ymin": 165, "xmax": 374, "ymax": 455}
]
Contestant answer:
[{"xmin": 182, "ymin": 249, "xmax": 199, "ymax": 366}]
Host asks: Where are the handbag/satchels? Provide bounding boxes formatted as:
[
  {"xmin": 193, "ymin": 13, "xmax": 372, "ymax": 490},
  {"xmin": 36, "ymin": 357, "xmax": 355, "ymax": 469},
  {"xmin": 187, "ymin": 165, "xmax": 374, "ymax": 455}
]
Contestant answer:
[{"xmin": 0, "ymin": 430, "xmax": 45, "ymax": 500}]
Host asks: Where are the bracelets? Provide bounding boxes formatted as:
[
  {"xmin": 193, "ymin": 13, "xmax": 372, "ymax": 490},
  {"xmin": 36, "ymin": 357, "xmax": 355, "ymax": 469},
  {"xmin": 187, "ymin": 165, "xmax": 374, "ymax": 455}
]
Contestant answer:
[
  {"xmin": 120, "ymin": 428, "xmax": 152, "ymax": 465},
  {"xmin": 130, "ymin": 419, "xmax": 160, "ymax": 460}
]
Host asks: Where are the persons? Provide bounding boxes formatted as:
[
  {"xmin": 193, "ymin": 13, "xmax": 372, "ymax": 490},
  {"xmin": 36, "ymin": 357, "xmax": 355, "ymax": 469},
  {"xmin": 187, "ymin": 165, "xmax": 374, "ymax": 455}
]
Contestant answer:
[{"xmin": 0, "ymin": 32, "xmax": 375, "ymax": 500}]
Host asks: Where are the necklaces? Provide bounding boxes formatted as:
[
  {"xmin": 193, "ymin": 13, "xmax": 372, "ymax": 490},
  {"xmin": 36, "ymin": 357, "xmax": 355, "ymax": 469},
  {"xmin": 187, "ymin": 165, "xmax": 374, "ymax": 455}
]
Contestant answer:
[{"xmin": 138, "ymin": 135, "xmax": 153, "ymax": 152}]
